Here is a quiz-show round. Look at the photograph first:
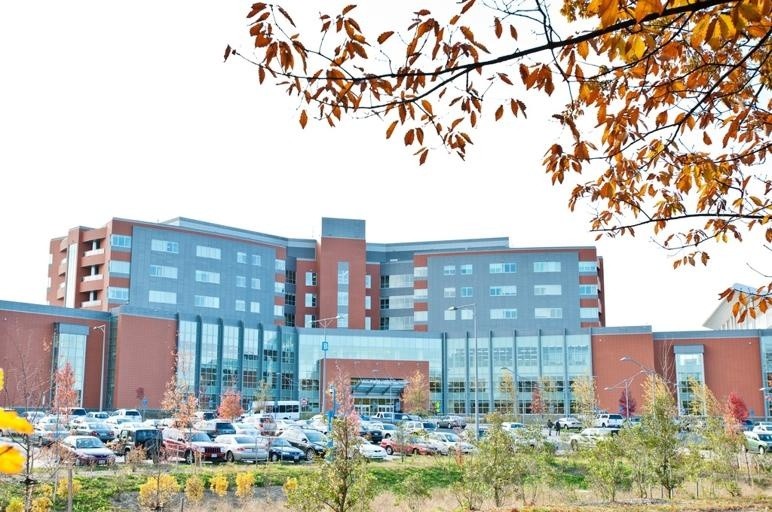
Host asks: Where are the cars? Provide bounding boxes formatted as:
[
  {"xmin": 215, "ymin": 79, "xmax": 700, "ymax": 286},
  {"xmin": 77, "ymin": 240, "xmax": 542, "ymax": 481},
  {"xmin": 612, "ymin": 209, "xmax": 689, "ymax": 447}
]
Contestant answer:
[
  {"xmin": 680, "ymin": 416, "xmax": 772, "ymax": 438},
  {"xmin": 627, "ymin": 415, "xmax": 642, "ymax": 425},
  {"xmin": 553, "ymin": 416, "xmax": 582, "ymax": 431}
]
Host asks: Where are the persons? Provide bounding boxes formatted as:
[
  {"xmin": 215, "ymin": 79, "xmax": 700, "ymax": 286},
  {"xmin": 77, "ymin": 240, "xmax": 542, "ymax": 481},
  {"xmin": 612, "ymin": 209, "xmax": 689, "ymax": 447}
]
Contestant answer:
[
  {"xmin": 547, "ymin": 419, "xmax": 553, "ymax": 436},
  {"xmin": 556, "ymin": 420, "xmax": 561, "ymax": 436}
]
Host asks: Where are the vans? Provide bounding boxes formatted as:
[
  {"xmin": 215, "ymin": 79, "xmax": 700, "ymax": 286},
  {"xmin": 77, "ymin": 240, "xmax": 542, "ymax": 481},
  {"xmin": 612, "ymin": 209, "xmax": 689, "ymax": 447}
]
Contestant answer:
[
  {"xmin": 568, "ymin": 427, "xmax": 622, "ymax": 453},
  {"xmin": 591, "ymin": 413, "xmax": 624, "ymax": 427},
  {"xmin": 742, "ymin": 431, "xmax": 772, "ymax": 455}
]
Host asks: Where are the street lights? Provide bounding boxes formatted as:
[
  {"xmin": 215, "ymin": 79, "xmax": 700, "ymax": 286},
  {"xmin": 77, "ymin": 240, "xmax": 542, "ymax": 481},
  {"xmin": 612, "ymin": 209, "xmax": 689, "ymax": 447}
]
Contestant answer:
[
  {"xmin": 618, "ymin": 356, "xmax": 657, "ymax": 435},
  {"xmin": 449, "ymin": 304, "xmax": 481, "ymax": 448},
  {"xmin": 604, "ymin": 385, "xmax": 629, "ymax": 418},
  {"xmin": 758, "ymin": 387, "xmax": 772, "ymax": 392},
  {"xmin": 327, "ymin": 384, "xmax": 336, "ymax": 415},
  {"xmin": 308, "ymin": 315, "xmax": 346, "ymax": 415},
  {"xmin": 92, "ymin": 322, "xmax": 108, "ymax": 414}
]
